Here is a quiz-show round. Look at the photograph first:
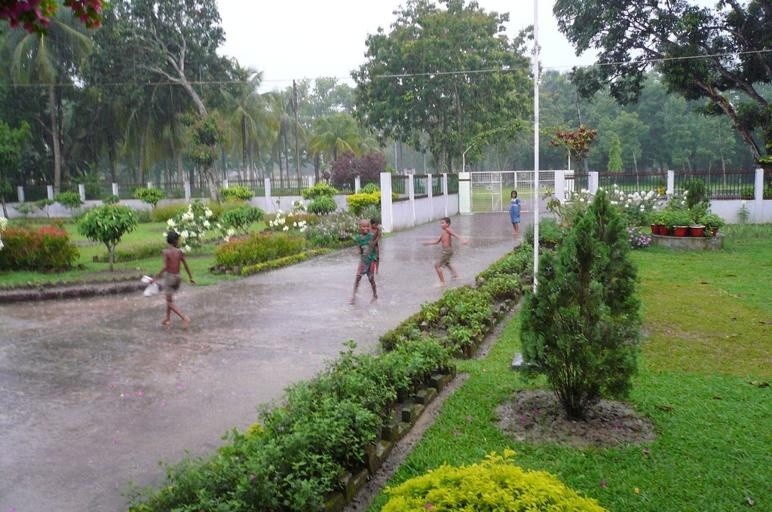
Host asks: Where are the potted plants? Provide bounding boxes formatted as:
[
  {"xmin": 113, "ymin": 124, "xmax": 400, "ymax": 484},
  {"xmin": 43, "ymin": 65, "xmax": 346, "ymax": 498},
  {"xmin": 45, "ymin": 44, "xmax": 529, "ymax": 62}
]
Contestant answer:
[{"xmin": 650, "ymin": 178, "xmax": 726, "ymax": 237}]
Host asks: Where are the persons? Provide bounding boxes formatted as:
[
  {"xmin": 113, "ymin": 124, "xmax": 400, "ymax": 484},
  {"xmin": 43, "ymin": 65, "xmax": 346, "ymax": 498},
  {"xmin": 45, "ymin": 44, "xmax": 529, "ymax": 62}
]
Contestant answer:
[
  {"xmin": 349, "ymin": 219, "xmax": 379, "ymax": 306},
  {"xmin": 370, "ymin": 219, "xmax": 381, "ymax": 273},
  {"xmin": 508, "ymin": 190, "xmax": 523, "ymax": 235},
  {"xmin": 148, "ymin": 231, "xmax": 197, "ymax": 330},
  {"xmin": 421, "ymin": 217, "xmax": 469, "ymax": 289}
]
